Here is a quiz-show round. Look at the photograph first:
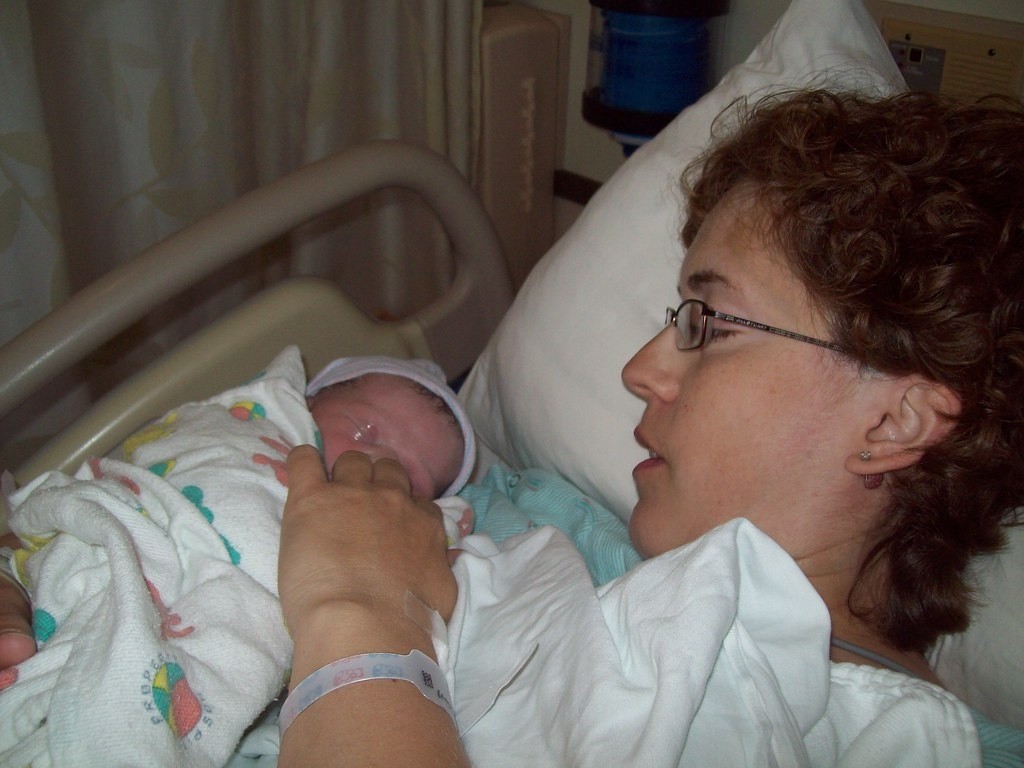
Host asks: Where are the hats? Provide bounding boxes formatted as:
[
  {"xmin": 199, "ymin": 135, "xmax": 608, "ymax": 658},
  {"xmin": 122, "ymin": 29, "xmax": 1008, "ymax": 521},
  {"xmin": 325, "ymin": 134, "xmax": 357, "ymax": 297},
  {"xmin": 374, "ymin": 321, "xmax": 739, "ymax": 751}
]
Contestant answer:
[{"xmin": 303, "ymin": 352, "xmax": 478, "ymax": 499}]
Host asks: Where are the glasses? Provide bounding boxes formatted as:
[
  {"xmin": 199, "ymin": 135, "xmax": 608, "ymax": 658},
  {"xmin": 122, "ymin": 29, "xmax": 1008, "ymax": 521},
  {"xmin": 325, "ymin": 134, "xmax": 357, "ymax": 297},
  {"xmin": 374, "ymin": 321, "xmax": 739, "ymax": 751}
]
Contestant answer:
[{"xmin": 665, "ymin": 300, "xmax": 850, "ymax": 351}]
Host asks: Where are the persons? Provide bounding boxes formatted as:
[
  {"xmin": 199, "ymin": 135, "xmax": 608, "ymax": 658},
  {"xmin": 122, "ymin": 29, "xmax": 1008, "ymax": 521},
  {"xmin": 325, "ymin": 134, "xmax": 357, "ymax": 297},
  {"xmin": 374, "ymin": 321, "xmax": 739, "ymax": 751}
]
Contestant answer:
[
  {"xmin": 1, "ymin": 341, "xmax": 476, "ymax": 767},
  {"xmin": 0, "ymin": 84, "xmax": 1024, "ymax": 767}
]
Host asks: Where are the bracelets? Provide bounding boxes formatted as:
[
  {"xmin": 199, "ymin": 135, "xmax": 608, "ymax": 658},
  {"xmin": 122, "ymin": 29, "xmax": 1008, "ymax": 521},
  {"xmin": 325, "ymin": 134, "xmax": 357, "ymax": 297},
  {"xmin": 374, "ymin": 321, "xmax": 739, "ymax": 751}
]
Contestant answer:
[{"xmin": 276, "ymin": 648, "xmax": 458, "ymax": 741}]
